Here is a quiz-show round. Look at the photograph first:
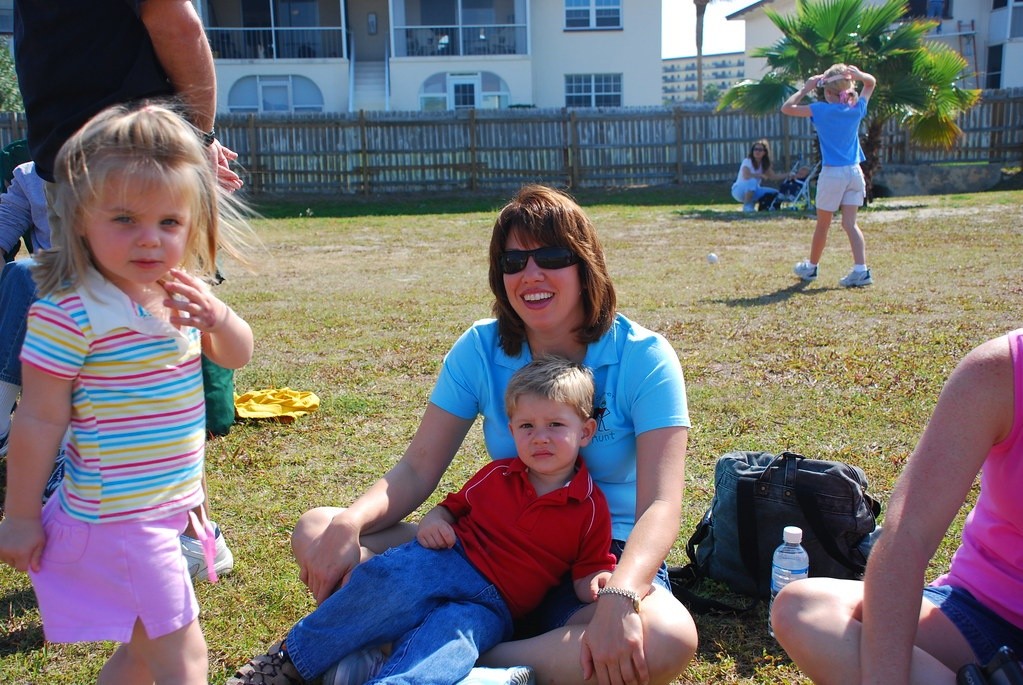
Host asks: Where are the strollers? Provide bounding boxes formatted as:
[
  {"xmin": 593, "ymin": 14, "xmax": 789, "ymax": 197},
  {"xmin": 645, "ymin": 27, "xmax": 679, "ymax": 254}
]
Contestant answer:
[{"xmin": 757, "ymin": 159, "xmax": 822, "ymax": 213}]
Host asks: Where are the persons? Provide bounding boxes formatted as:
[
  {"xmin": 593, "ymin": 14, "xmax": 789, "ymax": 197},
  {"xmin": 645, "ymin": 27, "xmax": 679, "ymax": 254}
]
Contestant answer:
[
  {"xmin": 731, "ymin": 139, "xmax": 789, "ymax": 213},
  {"xmin": 0, "ymin": 106, "xmax": 256, "ymax": 685},
  {"xmin": 225, "ymin": 354, "xmax": 618, "ymax": 685},
  {"xmin": 781, "ymin": 63, "xmax": 877, "ymax": 288},
  {"xmin": 0, "ymin": 0, "xmax": 243, "ymax": 502},
  {"xmin": 290, "ymin": 183, "xmax": 697, "ymax": 685},
  {"xmin": 771, "ymin": 329, "xmax": 1023, "ymax": 685},
  {"xmin": 779, "ymin": 166, "xmax": 810, "ymax": 197}
]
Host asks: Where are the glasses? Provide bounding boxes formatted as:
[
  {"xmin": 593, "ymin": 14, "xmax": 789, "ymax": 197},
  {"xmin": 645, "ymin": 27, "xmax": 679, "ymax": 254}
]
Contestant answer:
[
  {"xmin": 499, "ymin": 244, "xmax": 579, "ymax": 274},
  {"xmin": 753, "ymin": 148, "xmax": 766, "ymax": 152}
]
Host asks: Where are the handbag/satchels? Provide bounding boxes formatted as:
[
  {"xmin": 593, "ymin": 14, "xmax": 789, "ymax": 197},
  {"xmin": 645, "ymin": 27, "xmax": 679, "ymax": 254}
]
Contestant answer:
[{"xmin": 685, "ymin": 449, "xmax": 882, "ymax": 604}]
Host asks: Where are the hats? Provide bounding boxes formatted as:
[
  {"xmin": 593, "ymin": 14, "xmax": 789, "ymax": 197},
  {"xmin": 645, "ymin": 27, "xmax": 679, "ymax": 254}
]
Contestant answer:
[{"xmin": 816, "ymin": 73, "xmax": 853, "ymax": 88}]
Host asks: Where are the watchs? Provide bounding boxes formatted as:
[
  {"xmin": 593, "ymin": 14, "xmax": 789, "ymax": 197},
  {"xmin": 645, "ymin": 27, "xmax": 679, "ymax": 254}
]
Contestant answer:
[
  {"xmin": 597, "ymin": 587, "xmax": 641, "ymax": 613},
  {"xmin": 203, "ymin": 131, "xmax": 215, "ymax": 147}
]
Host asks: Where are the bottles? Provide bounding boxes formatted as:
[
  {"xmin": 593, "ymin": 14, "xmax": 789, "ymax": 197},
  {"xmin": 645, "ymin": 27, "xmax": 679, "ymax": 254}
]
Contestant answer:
[{"xmin": 768, "ymin": 527, "xmax": 809, "ymax": 639}]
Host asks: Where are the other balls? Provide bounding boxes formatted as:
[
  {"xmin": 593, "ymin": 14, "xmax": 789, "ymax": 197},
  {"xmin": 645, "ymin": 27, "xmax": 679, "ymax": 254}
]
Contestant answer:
[{"xmin": 708, "ymin": 253, "xmax": 718, "ymax": 263}]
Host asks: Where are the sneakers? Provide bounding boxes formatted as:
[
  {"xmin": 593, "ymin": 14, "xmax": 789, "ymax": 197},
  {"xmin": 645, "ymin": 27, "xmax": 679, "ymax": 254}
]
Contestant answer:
[
  {"xmin": 794, "ymin": 261, "xmax": 818, "ymax": 280},
  {"xmin": 319, "ymin": 643, "xmax": 390, "ymax": 684},
  {"xmin": 226, "ymin": 638, "xmax": 303, "ymax": 685},
  {"xmin": 839, "ymin": 268, "xmax": 872, "ymax": 286},
  {"xmin": 453, "ymin": 665, "xmax": 535, "ymax": 685},
  {"xmin": 742, "ymin": 203, "xmax": 760, "ymax": 218},
  {"xmin": 178, "ymin": 520, "xmax": 234, "ymax": 581}
]
